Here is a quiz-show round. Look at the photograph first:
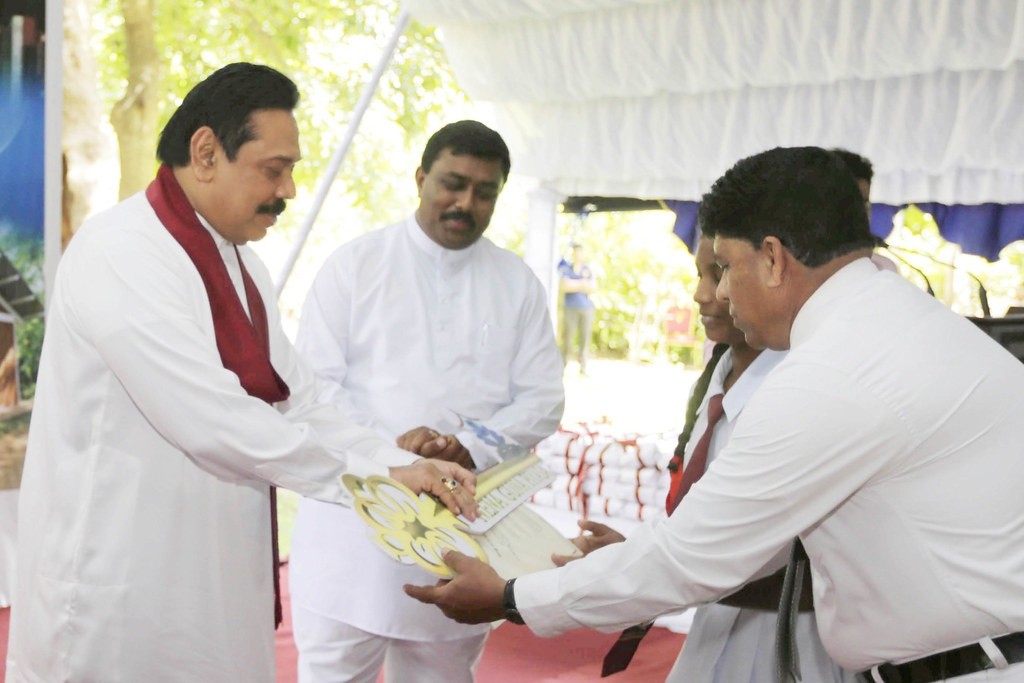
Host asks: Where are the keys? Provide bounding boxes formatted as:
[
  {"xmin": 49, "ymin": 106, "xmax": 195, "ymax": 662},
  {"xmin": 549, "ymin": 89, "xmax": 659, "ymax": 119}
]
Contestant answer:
[{"xmin": 343, "ymin": 452, "xmax": 554, "ymax": 580}]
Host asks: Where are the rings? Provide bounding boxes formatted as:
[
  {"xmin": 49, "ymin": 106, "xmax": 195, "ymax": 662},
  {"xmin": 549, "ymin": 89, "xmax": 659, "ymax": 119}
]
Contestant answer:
[
  {"xmin": 440, "ymin": 477, "xmax": 458, "ymax": 493},
  {"xmin": 430, "ymin": 429, "xmax": 439, "ymax": 438}
]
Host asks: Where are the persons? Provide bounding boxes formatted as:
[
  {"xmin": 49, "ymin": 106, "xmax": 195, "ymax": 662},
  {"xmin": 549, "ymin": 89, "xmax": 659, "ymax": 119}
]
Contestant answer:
[
  {"xmin": 830, "ymin": 148, "xmax": 897, "ymax": 270},
  {"xmin": 402, "ymin": 146, "xmax": 1024, "ymax": 683},
  {"xmin": 551, "ymin": 231, "xmax": 875, "ymax": 683},
  {"xmin": 4, "ymin": 63, "xmax": 482, "ymax": 683},
  {"xmin": 290, "ymin": 120, "xmax": 565, "ymax": 683},
  {"xmin": 560, "ymin": 243, "xmax": 595, "ymax": 373}
]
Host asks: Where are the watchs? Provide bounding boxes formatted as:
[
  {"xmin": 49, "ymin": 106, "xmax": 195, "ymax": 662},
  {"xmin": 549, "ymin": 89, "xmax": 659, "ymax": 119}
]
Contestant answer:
[{"xmin": 503, "ymin": 578, "xmax": 526, "ymax": 625}]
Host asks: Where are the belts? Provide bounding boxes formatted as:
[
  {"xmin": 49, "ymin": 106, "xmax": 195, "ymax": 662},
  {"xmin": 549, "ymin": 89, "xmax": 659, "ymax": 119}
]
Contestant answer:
[{"xmin": 863, "ymin": 631, "xmax": 1024, "ymax": 683}]
